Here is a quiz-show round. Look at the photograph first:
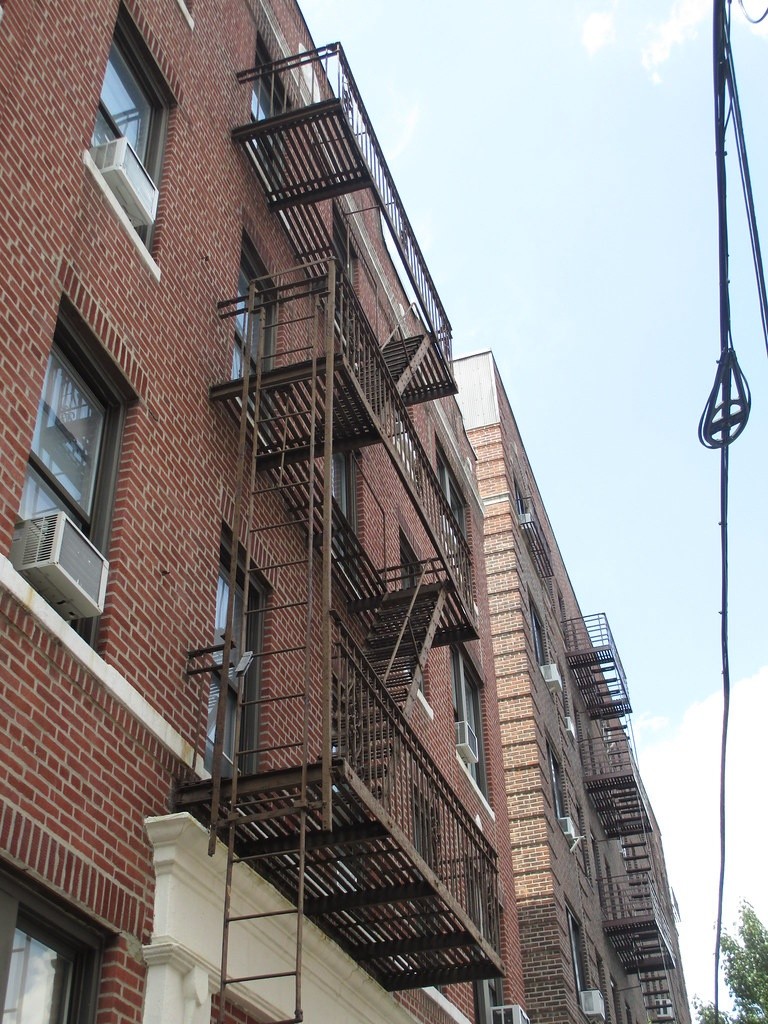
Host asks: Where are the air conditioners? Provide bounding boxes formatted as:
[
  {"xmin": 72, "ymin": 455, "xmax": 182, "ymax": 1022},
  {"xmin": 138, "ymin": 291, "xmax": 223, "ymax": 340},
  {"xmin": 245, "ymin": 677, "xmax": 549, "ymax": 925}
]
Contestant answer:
[
  {"xmin": 565, "ymin": 717, "xmax": 576, "ymax": 740},
  {"xmin": 655, "ymin": 999, "xmax": 674, "ymax": 1020},
  {"xmin": 540, "ymin": 663, "xmax": 563, "ymax": 693},
  {"xmin": 90, "ymin": 136, "xmax": 159, "ymax": 227},
  {"xmin": 454, "ymin": 721, "xmax": 478, "ymax": 765},
  {"xmin": 579, "ymin": 990, "xmax": 605, "ymax": 1020},
  {"xmin": 557, "ymin": 817, "xmax": 575, "ymax": 845},
  {"xmin": 9, "ymin": 510, "xmax": 110, "ymax": 622},
  {"xmin": 518, "ymin": 513, "xmax": 537, "ymax": 542},
  {"xmin": 490, "ymin": 1004, "xmax": 531, "ymax": 1024}
]
